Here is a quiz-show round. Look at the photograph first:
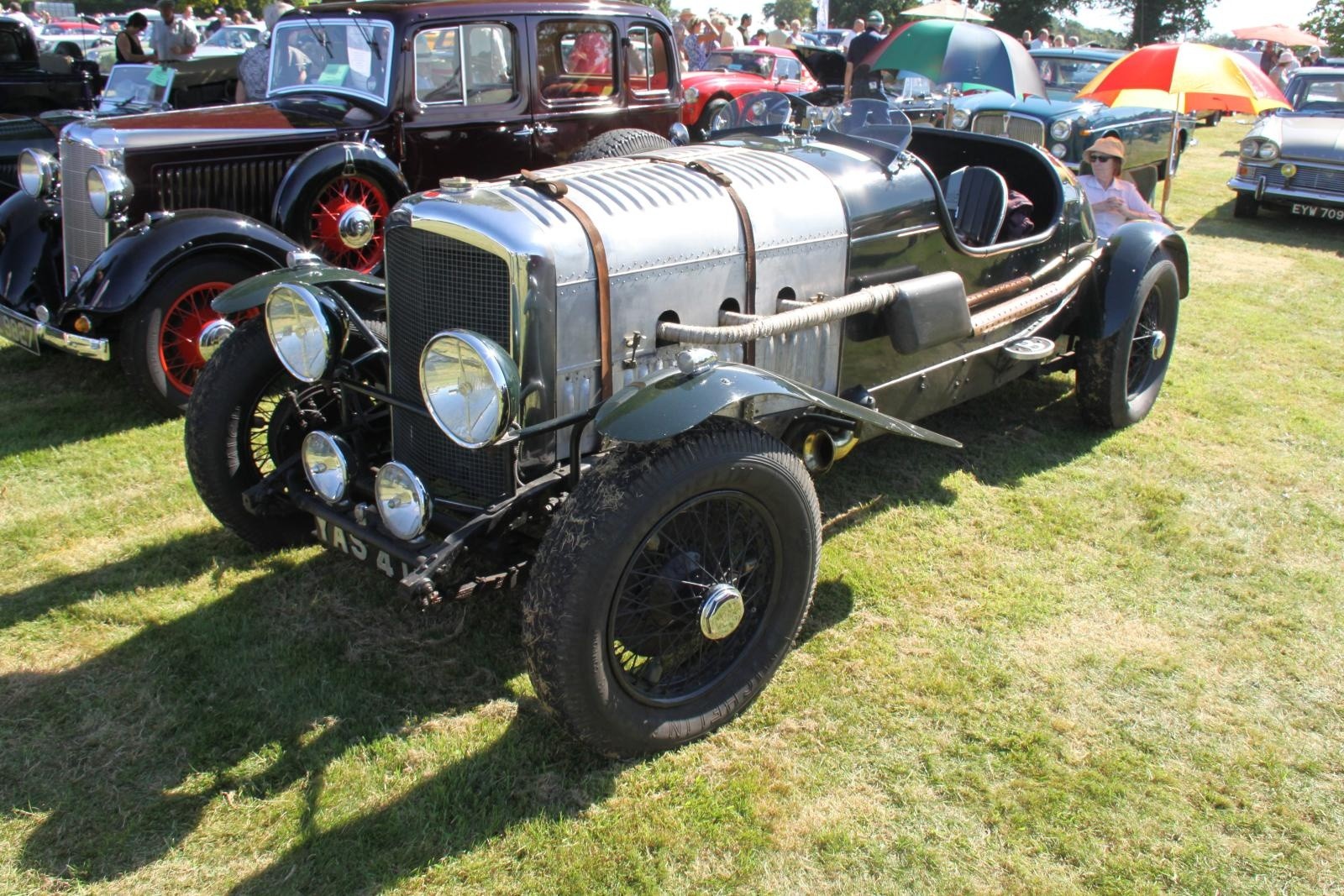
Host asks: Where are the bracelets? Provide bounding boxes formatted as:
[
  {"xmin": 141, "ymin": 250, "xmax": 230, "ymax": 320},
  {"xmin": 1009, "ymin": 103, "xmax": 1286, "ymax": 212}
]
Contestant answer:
[{"xmin": 844, "ymin": 91, "xmax": 851, "ymax": 94}]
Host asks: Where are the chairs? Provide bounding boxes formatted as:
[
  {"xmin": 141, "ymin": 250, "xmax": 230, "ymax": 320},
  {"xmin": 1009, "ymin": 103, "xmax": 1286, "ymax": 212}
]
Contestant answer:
[{"xmin": 938, "ymin": 166, "xmax": 1008, "ymax": 245}]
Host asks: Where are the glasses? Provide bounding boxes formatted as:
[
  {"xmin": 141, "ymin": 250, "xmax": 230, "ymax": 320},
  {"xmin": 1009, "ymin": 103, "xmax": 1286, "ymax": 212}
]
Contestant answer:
[{"xmin": 1090, "ymin": 155, "xmax": 1119, "ymax": 163}]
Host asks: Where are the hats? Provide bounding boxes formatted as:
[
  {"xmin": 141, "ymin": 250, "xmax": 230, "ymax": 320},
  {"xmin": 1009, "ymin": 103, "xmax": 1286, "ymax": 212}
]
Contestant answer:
[
  {"xmin": 10, "ymin": 2, "xmax": 22, "ymax": 11},
  {"xmin": 1279, "ymin": 52, "xmax": 1293, "ymax": 63},
  {"xmin": 708, "ymin": 7, "xmax": 718, "ymax": 15},
  {"xmin": 215, "ymin": 7, "xmax": 227, "ymax": 15},
  {"xmin": 264, "ymin": 3, "xmax": 297, "ymax": 30},
  {"xmin": 866, "ymin": 10, "xmax": 884, "ymax": 23},
  {"xmin": 1082, "ymin": 136, "xmax": 1126, "ymax": 170},
  {"xmin": 1267, "ymin": 41, "xmax": 1274, "ymax": 46},
  {"xmin": 677, "ymin": 8, "xmax": 696, "ymax": 17},
  {"xmin": 1309, "ymin": 46, "xmax": 1321, "ymax": 53},
  {"xmin": 155, "ymin": 0, "xmax": 175, "ymax": 8},
  {"xmin": 688, "ymin": 19, "xmax": 705, "ymax": 29}
]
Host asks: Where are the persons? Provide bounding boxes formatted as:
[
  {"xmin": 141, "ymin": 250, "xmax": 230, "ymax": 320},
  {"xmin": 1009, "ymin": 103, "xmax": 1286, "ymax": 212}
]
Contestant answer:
[
  {"xmin": 673, "ymin": 6, "xmax": 805, "ymax": 71},
  {"xmin": 469, "ymin": 26, "xmax": 510, "ymax": 83},
  {"xmin": 235, "ymin": 2, "xmax": 310, "ymax": 103},
  {"xmin": 1077, "ymin": 137, "xmax": 1162, "ymax": 239},
  {"xmin": 568, "ymin": 33, "xmax": 607, "ymax": 74},
  {"xmin": 1249, "ymin": 40, "xmax": 1327, "ymax": 85},
  {"xmin": 116, "ymin": 13, "xmax": 149, "ymax": 64},
  {"xmin": 842, "ymin": 10, "xmax": 892, "ymax": 130},
  {"xmin": 149, "ymin": 0, "xmax": 199, "ymax": 57},
  {"xmin": 6, "ymin": 2, "xmax": 33, "ymax": 28},
  {"xmin": 40, "ymin": 10, "xmax": 52, "ymax": 23},
  {"xmin": 1021, "ymin": 28, "xmax": 1079, "ymax": 69},
  {"xmin": 182, "ymin": 6, "xmax": 256, "ymax": 43}
]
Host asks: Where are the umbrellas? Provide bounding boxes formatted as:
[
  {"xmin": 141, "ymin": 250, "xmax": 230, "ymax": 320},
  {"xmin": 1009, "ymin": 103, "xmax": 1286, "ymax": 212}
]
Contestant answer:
[
  {"xmin": 1075, "ymin": 41, "xmax": 1295, "ymax": 214},
  {"xmin": 858, "ymin": 18, "xmax": 1051, "ymax": 126},
  {"xmin": 900, "ymin": 0, "xmax": 995, "ymax": 21},
  {"xmin": 1232, "ymin": 23, "xmax": 1329, "ymax": 52}
]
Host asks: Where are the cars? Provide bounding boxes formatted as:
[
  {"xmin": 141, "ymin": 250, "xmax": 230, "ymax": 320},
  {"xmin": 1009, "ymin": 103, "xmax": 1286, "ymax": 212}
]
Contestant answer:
[
  {"xmin": 1225, "ymin": 56, "xmax": 1344, "ymax": 228},
  {"xmin": 645, "ymin": 44, "xmax": 853, "ymax": 139},
  {"xmin": 183, "ymin": 93, "xmax": 1189, "ymax": 753},
  {"xmin": 0, "ymin": 0, "xmax": 682, "ymax": 412},
  {"xmin": 939, "ymin": 43, "xmax": 1193, "ymax": 182}
]
